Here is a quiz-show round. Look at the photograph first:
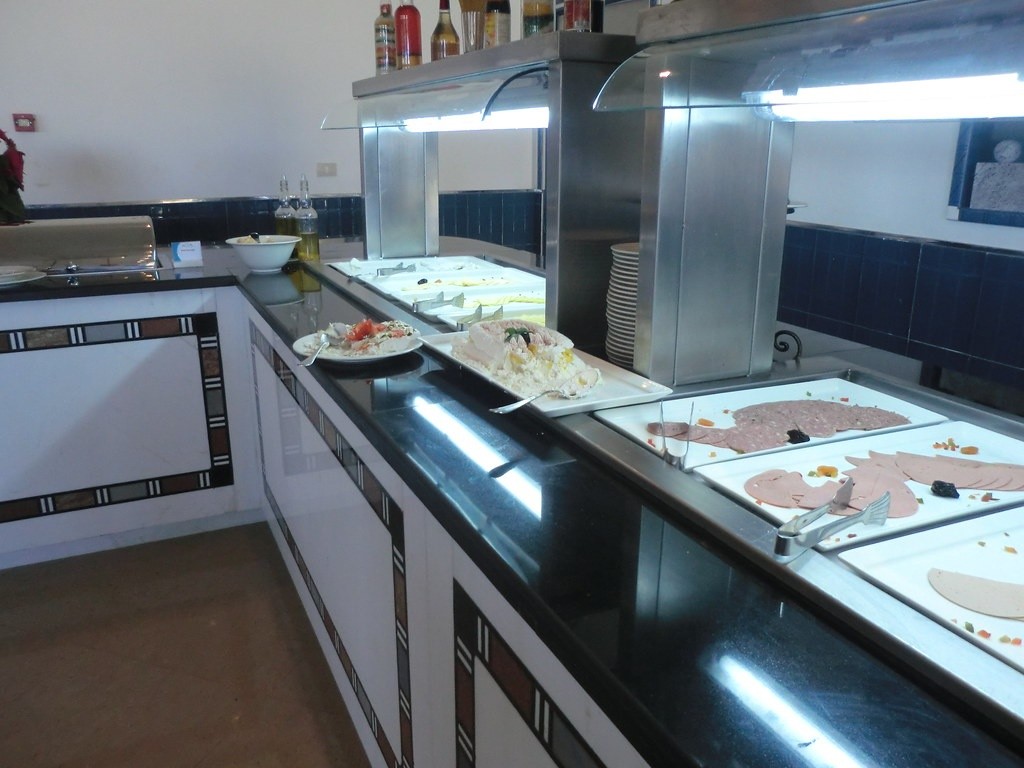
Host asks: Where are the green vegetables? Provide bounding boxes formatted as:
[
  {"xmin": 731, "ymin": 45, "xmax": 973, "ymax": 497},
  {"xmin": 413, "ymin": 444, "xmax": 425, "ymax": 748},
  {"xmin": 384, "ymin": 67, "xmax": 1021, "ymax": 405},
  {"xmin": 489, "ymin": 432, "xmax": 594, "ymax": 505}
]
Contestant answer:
[{"xmin": 504, "ymin": 326, "xmax": 536, "ymax": 346}]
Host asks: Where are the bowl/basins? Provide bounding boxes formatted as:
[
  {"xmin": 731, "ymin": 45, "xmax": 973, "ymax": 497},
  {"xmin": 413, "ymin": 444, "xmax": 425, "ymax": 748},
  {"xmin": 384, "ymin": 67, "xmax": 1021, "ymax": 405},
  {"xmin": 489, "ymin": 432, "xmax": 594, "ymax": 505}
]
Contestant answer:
[
  {"xmin": 241, "ymin": 272, "xmax": 306, "ymax": 308},
  {"xmin": 0, "ymin": 265, "xmax": 37, "ymax": 281},
  {"xmin": 226, "ymin": 235, "xmax": 302, "ymax": 272}
]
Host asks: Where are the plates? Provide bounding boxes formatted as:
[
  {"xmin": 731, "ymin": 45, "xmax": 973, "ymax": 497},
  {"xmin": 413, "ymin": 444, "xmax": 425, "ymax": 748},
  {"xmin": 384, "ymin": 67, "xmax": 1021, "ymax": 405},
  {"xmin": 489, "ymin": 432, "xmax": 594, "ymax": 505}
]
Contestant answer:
[
  {"xmin": 293, "ymin": 330, "xmax": 422, "ymax": 362},
  {"xmin": 604, "ymin": 242, "xmax": 643, "ymax": 364},
  {"xmin": 0, "ymin": 271, "xmax": 47, "ymax": 288},
  {"xmin": 330, "ymin": 254, "xmax": 545, "ymax": 330},
  {"xmin": 414, "ymin": 330, "xmax": 673, "ymax": 419},
  {"xmin": 315, "ymin": 351, "xmax": 424, "ymax": 382}
]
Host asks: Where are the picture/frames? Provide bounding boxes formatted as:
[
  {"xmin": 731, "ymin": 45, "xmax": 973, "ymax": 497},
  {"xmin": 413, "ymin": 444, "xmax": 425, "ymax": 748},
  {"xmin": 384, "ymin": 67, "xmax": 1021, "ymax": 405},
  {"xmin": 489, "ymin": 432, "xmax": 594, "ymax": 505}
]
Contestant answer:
[{"xmin": 944, "ymin": 118, "xmax": 1024, "ymax": 228}]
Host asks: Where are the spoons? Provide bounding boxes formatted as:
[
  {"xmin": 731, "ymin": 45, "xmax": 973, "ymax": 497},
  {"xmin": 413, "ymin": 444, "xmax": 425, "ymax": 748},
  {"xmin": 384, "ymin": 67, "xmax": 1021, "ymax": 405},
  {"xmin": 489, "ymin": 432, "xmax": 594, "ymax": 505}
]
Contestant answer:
[
  {"xmin": 296, "ymin": 322, "xmax": 347, "ymax": 367},
  {"xmin": 489, "ymin": 368, "xmax": 599, "ymax": 415}
]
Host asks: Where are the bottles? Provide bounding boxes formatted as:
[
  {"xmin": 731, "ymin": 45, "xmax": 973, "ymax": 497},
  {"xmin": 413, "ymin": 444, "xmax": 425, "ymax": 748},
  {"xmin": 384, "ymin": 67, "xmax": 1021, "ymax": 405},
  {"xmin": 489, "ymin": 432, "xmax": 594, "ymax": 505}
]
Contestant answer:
[
  {"xmin": 274, "ymin": 173, "xmax": 298, "ymax": 262},
  {"xmin": 430, "ymin": 0, "xmax": 460, "ymax": 61},
  {"xmin": 295, "ymin": 174, "xmax": 320, "ymax": 261},
  {"xmin": 520, "ymin": 0, "xmax": 554, "ymax": 39},
  {"xmin": 283, "ymin": 262, "xmax": 323, "ymax": 336},
  {"xmin": 483, "ymin": 0, "xmax": 511, "ymax": 51},
  {"xmin": 374, "ymin": 0, "xmax": 396, "ymax": 75},
  {"xmin": 393, "ymin": 0, "xmax": 422, "ymax": 71}
]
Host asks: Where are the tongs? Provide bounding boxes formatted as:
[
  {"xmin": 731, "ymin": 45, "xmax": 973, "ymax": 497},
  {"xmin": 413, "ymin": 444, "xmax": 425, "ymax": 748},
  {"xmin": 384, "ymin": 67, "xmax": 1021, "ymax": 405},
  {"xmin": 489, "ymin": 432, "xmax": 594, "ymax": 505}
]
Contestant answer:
[
  {"xmin": 456, "ymin": 304, "xmax": 504, "ymax": 332},
  {"xmin": 377, "ymin": 262, "xmax": 415, "ymax": 277},
  {"xmin": 412, "ymin": 291, "xmax": 465, "ymax": 314},
  {"xmin": 774, "ymin": 477, "xmax": 891, "ymax": 556},
  {"xmin": 659, "ymin": 399, "xmax": 695, "ymax": 468}
]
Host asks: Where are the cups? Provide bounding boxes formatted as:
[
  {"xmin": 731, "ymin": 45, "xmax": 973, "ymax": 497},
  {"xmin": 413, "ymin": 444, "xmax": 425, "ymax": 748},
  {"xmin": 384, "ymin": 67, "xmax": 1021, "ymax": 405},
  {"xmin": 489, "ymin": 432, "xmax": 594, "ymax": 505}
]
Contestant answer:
[
  {"xmin": 563, "ymin": 0, "xmax": 592, "ymax": 33},
  {"xmin": 461, "ymin": 12, "xmax": 484, "ymax": 54}
]
